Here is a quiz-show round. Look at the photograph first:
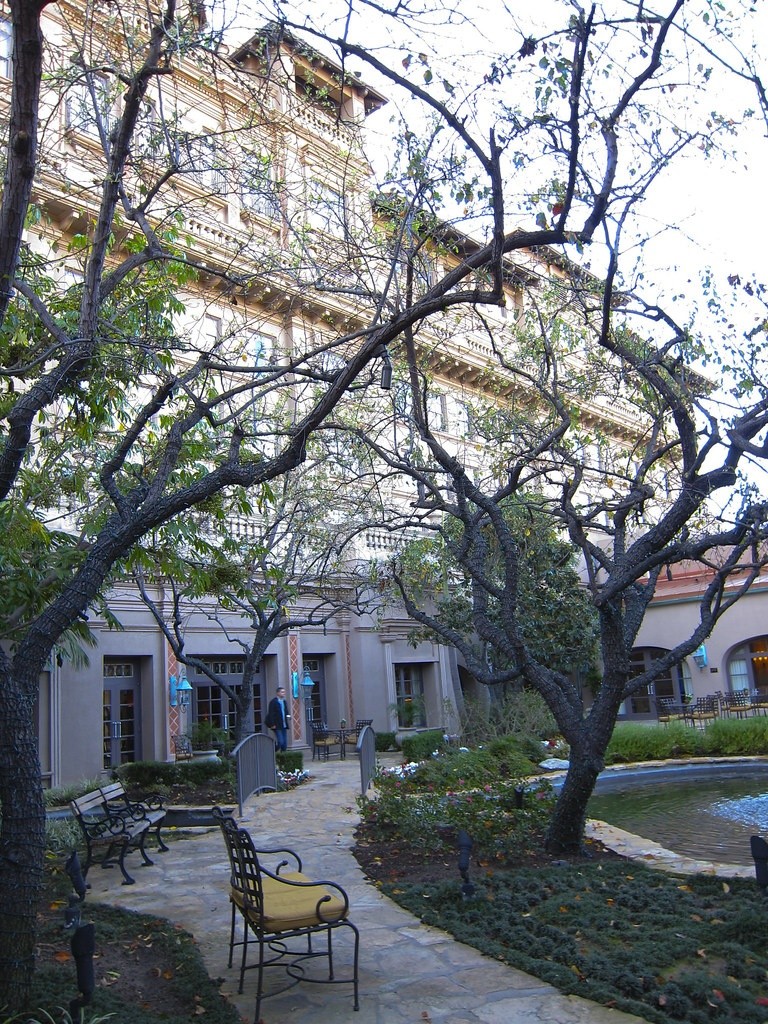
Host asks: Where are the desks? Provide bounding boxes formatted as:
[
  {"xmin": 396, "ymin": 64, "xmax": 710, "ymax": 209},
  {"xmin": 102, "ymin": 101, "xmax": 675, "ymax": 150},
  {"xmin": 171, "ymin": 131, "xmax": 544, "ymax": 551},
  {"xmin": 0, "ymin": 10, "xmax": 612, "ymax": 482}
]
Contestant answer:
[
  {"xmin": 325, "ymin": 728, "xmax": 358, "ymax": 761},
  {"xmin": 667, "ymin": 703, "xmax": 698, "ymax": 728}
]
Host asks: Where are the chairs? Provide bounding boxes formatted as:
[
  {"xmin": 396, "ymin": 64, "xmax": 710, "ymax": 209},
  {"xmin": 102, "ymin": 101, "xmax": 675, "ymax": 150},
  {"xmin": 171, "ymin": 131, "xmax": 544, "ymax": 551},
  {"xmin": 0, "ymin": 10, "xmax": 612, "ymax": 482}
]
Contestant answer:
[
  {"xmin": 171, "ymin": 734, "xmax": 194, "ymax": 764},
  {"xmin": 309, "ymin": 720, "xmax": 373, "ymax": 761},
  {"xmin": 656, "ymin": 688, "xmax": 768, "ymax": 731}
]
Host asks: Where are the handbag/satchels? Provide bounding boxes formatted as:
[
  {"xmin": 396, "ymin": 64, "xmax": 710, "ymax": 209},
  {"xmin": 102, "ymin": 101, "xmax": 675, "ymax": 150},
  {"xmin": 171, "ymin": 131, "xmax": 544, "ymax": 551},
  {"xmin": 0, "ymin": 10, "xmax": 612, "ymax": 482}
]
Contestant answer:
[{"xmin": 264, "ymin": 714, "xmax": 271, "ymax": 728}]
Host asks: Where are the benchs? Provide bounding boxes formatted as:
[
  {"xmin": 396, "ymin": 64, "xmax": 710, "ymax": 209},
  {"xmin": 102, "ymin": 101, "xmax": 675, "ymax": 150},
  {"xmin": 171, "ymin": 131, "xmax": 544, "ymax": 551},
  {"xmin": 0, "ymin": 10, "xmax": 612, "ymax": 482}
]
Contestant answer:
[
  {"xmin": 70, "ymin": 779, "xmax": 170, "ymax": 885},
  {"xmin": 209, "ymin": 805, "xmax": 359, "ymax": 1024}
]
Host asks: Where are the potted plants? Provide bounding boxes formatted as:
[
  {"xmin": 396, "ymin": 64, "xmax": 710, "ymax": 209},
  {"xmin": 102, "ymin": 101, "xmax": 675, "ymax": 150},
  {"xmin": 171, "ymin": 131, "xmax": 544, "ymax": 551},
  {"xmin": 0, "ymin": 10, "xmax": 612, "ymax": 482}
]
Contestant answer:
[
  {"xmin": 389, "ymin": 699, "xmax": 422, "ymax": 748},
  {"xmin": 186, "ymin": 721, "xmax": 238, "ymax": 766}
]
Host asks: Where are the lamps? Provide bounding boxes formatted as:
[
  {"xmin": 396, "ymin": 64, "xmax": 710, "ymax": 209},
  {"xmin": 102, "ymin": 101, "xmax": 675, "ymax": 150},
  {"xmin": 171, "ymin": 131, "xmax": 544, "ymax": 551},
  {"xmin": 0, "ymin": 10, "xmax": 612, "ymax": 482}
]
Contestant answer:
[
  {"xmin": 292, "ymin": 666, "xmax": 315, "ymax": 699},
  {"xmin": 693, "ymin": 645, "xmax": 707, "ymax": 667},
  {"xmin": 170, "ymin": 675, "xmax": 193, "ymax": 713}
]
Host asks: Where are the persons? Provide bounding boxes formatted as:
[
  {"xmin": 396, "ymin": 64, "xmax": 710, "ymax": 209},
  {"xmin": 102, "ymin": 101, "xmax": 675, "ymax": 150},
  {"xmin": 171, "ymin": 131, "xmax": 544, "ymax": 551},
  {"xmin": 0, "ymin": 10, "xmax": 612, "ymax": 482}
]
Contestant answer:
[{"xmin": 269, "ymin": 687, "xmax": 290, "ymax": 753}]
